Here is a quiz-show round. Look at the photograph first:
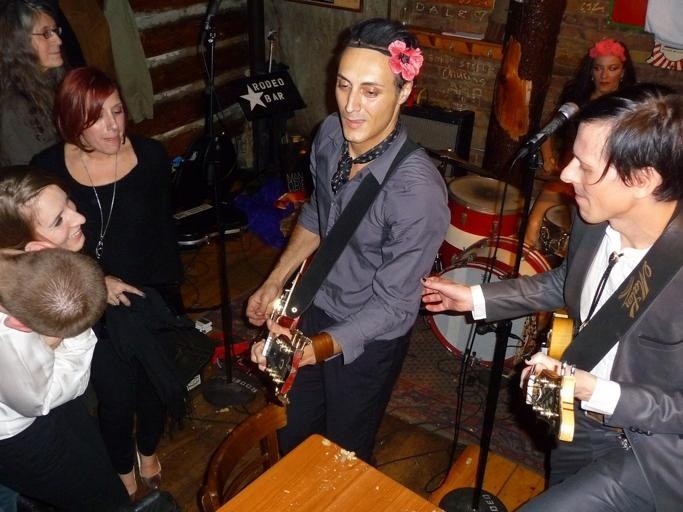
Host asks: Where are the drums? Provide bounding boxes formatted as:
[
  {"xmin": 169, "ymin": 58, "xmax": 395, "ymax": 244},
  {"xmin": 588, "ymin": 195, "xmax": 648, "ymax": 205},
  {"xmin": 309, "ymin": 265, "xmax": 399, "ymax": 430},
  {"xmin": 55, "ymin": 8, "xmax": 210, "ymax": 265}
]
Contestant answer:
[
  {"xmin": 424, "ymin": 236, "xmax": 554, "ymax": 370},
  {"xmin": 433, "ymin": 174, "xmax": 524, "ymax": 271},
  {"xmin": 539, "ymin": 205, "xmax": 570, "ymax": 260}
]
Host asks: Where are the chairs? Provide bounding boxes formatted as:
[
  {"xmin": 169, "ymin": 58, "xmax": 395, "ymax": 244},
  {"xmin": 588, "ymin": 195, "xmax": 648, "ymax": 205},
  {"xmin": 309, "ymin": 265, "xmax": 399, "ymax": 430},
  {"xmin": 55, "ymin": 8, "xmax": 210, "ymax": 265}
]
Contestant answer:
[{"xmin": 195, "ymin": 402, "xmax": 289, "ymax": 511}]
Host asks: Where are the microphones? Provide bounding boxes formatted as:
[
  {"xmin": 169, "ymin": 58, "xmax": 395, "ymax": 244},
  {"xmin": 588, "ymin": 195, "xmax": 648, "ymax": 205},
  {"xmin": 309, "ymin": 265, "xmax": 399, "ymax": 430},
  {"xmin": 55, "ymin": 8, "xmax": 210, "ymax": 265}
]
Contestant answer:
[
  {"xmin": 202, "ymin": 0, "xmax": 222, "ymax": 34},
  {"xmin": 516, "ymin": 101, "xmax": 581, "ymax": 160}
]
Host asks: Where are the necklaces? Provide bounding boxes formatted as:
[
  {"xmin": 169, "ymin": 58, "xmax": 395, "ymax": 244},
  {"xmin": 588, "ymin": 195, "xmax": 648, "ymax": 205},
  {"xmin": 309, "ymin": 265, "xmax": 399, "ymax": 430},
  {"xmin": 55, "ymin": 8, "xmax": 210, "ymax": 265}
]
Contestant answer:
[{"xmin": 72, "ymin": 141, "xmax": 120, "ymax": 261}]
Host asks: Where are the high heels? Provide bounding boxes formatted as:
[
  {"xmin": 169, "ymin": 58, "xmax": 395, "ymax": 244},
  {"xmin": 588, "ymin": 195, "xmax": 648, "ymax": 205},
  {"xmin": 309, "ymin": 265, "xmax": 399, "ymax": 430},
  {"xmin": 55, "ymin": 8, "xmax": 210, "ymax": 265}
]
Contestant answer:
[
  {"xmin": 133, "ymin": 433, "xmax": 162, "ymax": 492},
  {"xmin": 129, "ymin": 464, "xmax": 138, "ymax": 507}
]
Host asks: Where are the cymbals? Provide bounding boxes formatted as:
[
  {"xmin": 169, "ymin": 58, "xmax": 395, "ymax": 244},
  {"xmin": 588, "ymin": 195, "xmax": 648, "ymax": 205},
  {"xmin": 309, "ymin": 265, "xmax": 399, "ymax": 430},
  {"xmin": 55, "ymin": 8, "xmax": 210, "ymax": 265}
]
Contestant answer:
[{"xmin": 418, "ymin": 142, "xmax": 501, "ymax": 179}]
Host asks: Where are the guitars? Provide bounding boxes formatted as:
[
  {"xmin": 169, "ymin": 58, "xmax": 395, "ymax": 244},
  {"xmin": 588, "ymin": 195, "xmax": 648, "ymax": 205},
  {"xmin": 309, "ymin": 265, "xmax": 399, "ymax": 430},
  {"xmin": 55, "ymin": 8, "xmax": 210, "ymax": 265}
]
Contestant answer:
[
  {"xmin": 513, "ymin": 304, "xmax": 575, "ymax": 444},
  {"xmin": 259, "ymin": 255, "xmax": 313, "ymax": 394}
]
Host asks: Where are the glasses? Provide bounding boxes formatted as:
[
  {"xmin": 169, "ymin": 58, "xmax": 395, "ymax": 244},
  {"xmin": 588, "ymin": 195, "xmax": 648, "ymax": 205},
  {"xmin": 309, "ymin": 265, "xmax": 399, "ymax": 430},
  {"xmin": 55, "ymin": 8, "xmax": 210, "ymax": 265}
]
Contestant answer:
[{"xmin": 31, "ymin": 26, "xmax": 62, "ymax": 40}]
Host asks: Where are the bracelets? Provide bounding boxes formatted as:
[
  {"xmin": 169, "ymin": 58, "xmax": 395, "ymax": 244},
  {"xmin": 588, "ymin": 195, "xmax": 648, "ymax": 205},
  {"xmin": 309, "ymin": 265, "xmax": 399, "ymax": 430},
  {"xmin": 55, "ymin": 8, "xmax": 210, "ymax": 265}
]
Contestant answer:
[{"xmin": 307, "ymin": 329, "xmax": 335, "ymax": 370}]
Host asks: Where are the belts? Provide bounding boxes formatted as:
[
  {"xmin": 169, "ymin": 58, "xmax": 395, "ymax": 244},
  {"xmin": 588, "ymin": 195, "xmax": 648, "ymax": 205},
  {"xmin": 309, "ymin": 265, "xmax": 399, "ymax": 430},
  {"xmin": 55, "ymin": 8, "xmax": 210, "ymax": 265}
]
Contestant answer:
[{"xmin": 584, "ymin": 409, "xmax": 623, "ymax": 432}]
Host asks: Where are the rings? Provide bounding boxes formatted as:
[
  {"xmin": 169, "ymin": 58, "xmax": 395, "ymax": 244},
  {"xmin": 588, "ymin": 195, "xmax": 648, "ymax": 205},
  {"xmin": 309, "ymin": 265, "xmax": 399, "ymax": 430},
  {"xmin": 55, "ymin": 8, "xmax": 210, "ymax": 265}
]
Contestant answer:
[{"xmin": 114, "ymin": 292, "xmax": 122, "ymax": 298}]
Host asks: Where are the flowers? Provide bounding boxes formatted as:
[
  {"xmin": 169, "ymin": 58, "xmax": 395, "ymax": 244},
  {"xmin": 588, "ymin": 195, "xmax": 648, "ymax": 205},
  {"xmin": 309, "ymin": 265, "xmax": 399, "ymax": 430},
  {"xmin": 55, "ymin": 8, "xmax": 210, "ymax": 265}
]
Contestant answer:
[{"xmin": 386, "ymin": 38, "xmax": 427, "ymax": 83}]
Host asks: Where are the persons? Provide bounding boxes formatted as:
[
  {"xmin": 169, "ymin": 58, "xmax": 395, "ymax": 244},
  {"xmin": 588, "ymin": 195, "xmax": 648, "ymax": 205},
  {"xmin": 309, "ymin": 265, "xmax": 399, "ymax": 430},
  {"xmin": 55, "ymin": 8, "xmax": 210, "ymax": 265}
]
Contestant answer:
[
  {"xmin": 547, "ymin": 36, "xmax": 638, "ymax": 178},
  {"xmin": 23, "ymin": 63, "xmax": 190, "ymax": 505},
  {"xmin": 0, "ymin": 0, "xmax": 71, "ymax": 169},
  {"xmin": 243, "ymin": 16, "xmax": 450, "ymax": 467},
  {"xmin": 418, "ymin": 80, "xmax": 683, "ymax": 511},
  {"xmin": 0, "ymin": 164, "xmax": 137, "ymax": 511},
  {"xmin": 1, "ymin": 236, "xmax": 105, "ymax": 339}
]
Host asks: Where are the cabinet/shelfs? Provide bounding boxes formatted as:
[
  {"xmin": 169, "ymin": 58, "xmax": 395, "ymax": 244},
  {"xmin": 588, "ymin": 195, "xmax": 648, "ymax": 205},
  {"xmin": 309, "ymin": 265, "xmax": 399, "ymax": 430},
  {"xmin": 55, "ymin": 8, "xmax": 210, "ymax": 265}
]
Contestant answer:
[{"xmin": 399, "ymin": 102, "xmax": 475, "ymax": 176}]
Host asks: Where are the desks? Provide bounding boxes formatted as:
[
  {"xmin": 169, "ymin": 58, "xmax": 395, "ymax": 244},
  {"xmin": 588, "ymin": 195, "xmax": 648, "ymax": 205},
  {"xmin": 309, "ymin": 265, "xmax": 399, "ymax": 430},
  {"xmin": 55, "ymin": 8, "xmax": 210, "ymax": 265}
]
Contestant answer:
[{"xmin": 212, "ymin": 431, "xmax": 450, "ymax": 512}]
